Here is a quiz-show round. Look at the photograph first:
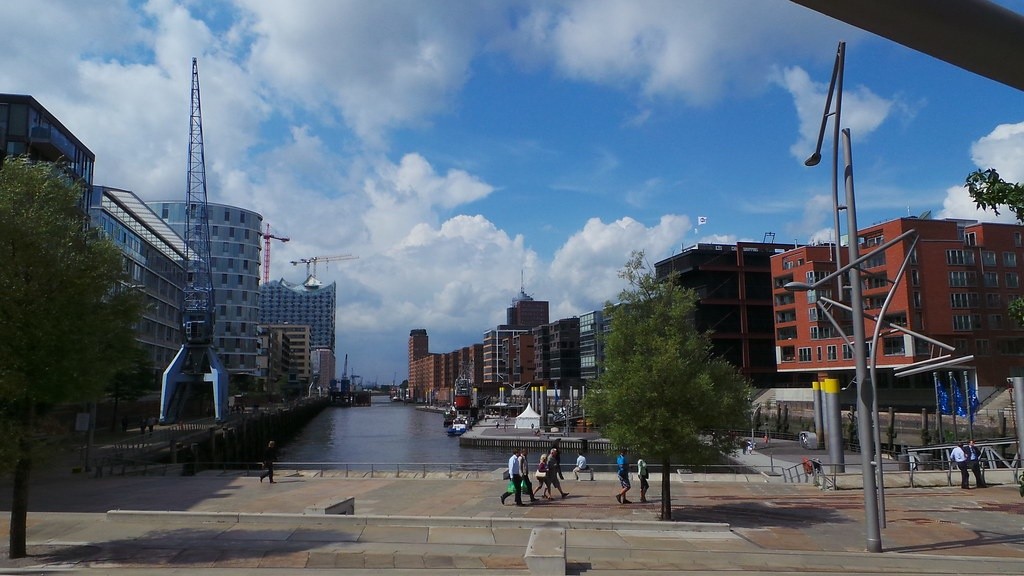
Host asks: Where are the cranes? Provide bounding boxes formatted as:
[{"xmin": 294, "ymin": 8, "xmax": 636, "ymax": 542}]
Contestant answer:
[
  {"xmin": 290, "ymin": 257, "xmax": 360, "ymax": 281},
  {"xmin": 342, "ymin": 352, "xmax": 398, "ymax": 387},
  {"xmin": 157, "ymin": 60, "xmax": 230, "ymax": 425},
  {"xmin": 262, "ymin": 221, "xmax": 291, "ymax": 283}
]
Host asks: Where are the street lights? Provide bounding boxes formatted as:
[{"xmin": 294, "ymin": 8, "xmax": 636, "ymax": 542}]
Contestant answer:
[{"xmin": 784, "ymin": 38, "xmax": 975, "ymax": 554}]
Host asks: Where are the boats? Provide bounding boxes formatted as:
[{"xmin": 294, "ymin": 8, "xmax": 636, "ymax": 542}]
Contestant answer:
[
  {"xmin": 444, "ymin": 374, "xmax": 477, "ymax": 426},
  {"xmin": 446, "ymin": 416, "xmax": 472, "ymax": 435}
]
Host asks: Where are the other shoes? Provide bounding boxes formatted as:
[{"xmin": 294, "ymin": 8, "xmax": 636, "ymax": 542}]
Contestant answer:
[
  {"xmin": 271, "ymin": 482, "xmax": 276, "ymax": 483},
  {"xmin": 531, "ymin": 499, "xmax": 539, "ymax": 502},
  {"xmin": 542, "ymin": 495, "xmax": 547, "ymax": 498},
  {"xmin": 548, "ymin": 497, "xmax": 554, "ymax": 500},
  {"xmin": 501, "ymin": 496, "xmax": 504, "ymax": 505},
  {"xmin": 562, "ymin": 493, "xmax": 569, "ymax": 498},
  {"xmin": 259, "ymin": 477, "xmax": 262, "ymax": 483},
  {"xmin": 623, "ymin": 500, "xmax": 632, "ymax": 504},
  {"xmin": 616, "ymin": 495, "xmax": 622, "ymax": 503}
]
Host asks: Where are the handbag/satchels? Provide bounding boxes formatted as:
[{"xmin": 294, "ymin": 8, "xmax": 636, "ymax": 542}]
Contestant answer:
[
  {"xmin": 507, "ymin": 479, "xmax": 516, "ymax": 493},
  {"xmin": 536, "ymin": 469, "xmax": 547, "ymax": 479},
  {"xmin": 521, "ymin": 480, "xmax": 528, "ymax": 493}
]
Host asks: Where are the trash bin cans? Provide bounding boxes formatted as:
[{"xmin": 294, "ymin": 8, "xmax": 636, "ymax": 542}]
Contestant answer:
[
  {"xmin": 804, "ymin": 433, "xmax": 818, "ymax": 449},
  {"xmin": 799, "ymin": 430, "xmax": 809, "ymax": 445},
  {"xmin": 919, "ymin": 453, "xmax": 935, "ymax": 469},
  {"xmin": 898, "ymin": 454, "xmax": 910, "ymax": 470}
]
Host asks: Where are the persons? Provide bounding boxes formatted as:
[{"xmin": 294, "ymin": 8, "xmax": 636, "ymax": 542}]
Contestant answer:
[
  {"xmin": 573, "ymin": 450, "xmax": 587, "ymax": 480},
  {"xmin": 950, "ymin": 442, "xmax": 969, "ymax": 489},
  {"xmin": 615, "ymin": 449, "xmax": 633, "ymax": 504},
  {"xmin": 638, "ymin": 452, "xmax": 649, "ymax": 503},
  {"xmin": 547, "ymin": 440, "xmax": 564, "ymax": 479},
  {"xmin": 140, "ymin": 416, "xmax": 147, "ymax": 434},
  {"xmin": 748, "ymin": 439, "xmax": 753, "ymax": 455},
  {"xmin": 741, "ymin": 438, "xmax": 748, "ymax": 454},
  {"xmin": 532, "ymin": 454, "xmax": 555, "ymax": 500},
  {"xmin": 147, "ymin": 414, "xmax": 155, "ymax": 438},
  {"xmin": 500, "ymin": 448, "xmax": 526, "ymax": 507},
  {"xmin": 122, "ymin": 416, "xmax": 128, "ymax": 432},
  {"xmin": 517, "ymin": 447, "xmax": 539, "ymax": 502},
  {"xmin": 965, "ymin": 439, "xmax": 988, "ymax": 488},
  {"xmin": 229, "ymin": 406, "xmax": 245, "ymax": 414},
  {"xmin": 542, "ymin": 448, "xmax": 569, "ymax": 498},
  {"xmin": 260, "ymin": 441, "xmax": 277, "ymax": 483}
]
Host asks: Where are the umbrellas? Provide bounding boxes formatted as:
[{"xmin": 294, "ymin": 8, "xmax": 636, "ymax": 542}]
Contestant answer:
[
  {"xmin": 937, "ymin": 379, "xmax": 951, "ymax": 415},
  {"xmin": 953, "ymin": 377, "xmax": 965, "ymax": 418},
  {"xmin": 967, "ymin": 379, "xmax": 980, "ymax": 423}
]
